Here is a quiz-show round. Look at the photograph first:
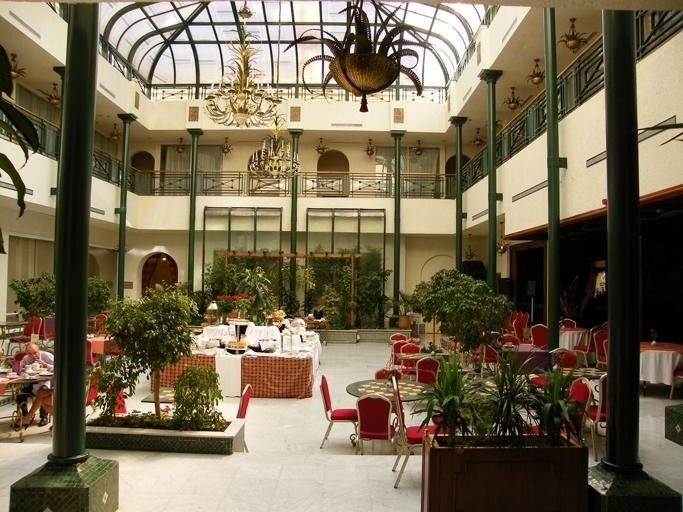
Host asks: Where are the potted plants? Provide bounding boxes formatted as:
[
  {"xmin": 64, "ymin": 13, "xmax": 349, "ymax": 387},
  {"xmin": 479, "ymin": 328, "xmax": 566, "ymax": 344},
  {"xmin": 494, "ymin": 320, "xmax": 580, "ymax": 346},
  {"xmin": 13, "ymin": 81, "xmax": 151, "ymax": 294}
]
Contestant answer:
[{"xmin": 419, "ymin": 354, "xmax": 592, "ymax": 512}]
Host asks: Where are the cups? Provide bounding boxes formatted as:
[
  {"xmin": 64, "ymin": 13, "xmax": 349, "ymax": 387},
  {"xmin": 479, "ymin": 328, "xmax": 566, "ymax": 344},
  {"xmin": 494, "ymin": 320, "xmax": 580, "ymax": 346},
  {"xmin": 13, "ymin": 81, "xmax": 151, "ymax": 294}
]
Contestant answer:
[
  {"xmin": 505, "ymin": 341, "xmax": 513, "ymax": 346},
  {"xmin": 7, "ymin": 372, "xmax": 17, "ymax": 377},
  {"xmin": 24, "ymin": 364, "xmax": 31, "ymax": 372},
  {"xmin": 32, "ymin": 361, "xmax": 44, "ymax": 370}
]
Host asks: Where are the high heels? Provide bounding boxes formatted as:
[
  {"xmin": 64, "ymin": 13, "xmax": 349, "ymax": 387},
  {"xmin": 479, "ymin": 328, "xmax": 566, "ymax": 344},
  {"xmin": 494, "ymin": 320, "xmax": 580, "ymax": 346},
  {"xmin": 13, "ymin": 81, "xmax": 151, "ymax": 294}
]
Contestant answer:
[{"xmin": 9, "ymin": 423, "xmax": 29, "ymax": 430}]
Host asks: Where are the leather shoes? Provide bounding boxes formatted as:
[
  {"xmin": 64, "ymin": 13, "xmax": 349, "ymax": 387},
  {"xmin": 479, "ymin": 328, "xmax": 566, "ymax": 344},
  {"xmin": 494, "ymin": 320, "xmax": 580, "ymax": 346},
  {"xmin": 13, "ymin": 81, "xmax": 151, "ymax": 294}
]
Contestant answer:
[{"xmin": 38, "ymin": 417, "xmax": 48, "ymax": 426}]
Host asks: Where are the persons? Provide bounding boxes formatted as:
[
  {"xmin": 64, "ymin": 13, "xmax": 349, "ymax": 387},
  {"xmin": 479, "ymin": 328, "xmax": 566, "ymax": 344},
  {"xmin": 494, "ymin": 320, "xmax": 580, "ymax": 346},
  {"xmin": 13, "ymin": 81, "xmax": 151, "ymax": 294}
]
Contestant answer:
[
  {"xmin": 14, "ymin": 368, "xmax": 91, "ymax": 431},
  {"xmin": 15, "ymin": 343, "xmax": 53, "ymax": 427}
]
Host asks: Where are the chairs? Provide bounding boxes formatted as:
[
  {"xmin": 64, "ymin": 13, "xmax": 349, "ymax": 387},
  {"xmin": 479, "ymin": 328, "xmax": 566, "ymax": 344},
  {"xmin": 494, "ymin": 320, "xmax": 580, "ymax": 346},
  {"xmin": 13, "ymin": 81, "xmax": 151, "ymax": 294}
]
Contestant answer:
[
  {"xmin": 318, "ymin": 312, "xmax": 683, "ymax": 489},
  {"xmin": 1, "ymin": 306, "xmax": 252, "ymax": 445}
]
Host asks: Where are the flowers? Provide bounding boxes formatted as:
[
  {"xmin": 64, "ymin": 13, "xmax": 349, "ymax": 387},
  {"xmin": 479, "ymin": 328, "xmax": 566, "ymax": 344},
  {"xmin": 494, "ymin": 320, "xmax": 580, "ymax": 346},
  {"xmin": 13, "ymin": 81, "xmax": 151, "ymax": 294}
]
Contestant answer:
[{"xmin": 216, "ymin": 294, "xmax": 254, "ymax": 304}]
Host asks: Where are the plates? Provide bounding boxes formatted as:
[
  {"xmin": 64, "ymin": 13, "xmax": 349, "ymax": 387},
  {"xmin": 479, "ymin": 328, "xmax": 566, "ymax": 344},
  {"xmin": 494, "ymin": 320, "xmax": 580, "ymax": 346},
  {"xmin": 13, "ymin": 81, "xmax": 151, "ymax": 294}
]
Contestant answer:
[
  {"xmin": 39, "ymin": 372, "xmax": 54, "ymax": 376},
  {"xmin": 6, "ymin": 376, "xmax": 20, "ymax": 379},
  {"xmin": 504, "ymin": 345, "xmax": 514, "ymax": 348}
]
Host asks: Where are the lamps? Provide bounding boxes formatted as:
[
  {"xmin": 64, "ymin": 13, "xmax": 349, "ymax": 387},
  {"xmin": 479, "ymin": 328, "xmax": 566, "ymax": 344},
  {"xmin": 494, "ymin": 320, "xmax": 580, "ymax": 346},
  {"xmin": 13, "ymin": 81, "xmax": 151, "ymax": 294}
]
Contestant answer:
[
  {"xmin": 202, "ymin": 0, "xmax": 303, "ymax": 179},
  {"xmin": 9, "ymin": 56, "xmax": 122, "ymax": 142},
  {"xmin": 463, "ymin": 222, "xmax": 510, "ymax": 260},
  {"xmin": 175, "ymin": 133, "xmax": 422, "ymax": 160},
  {"xmin": 288, "ymin": 1, "xmax": 425, "ymax": 111},
  {"xmin": 473, "ymin": 17, "xmax": 586, "ymax": 148}
]
